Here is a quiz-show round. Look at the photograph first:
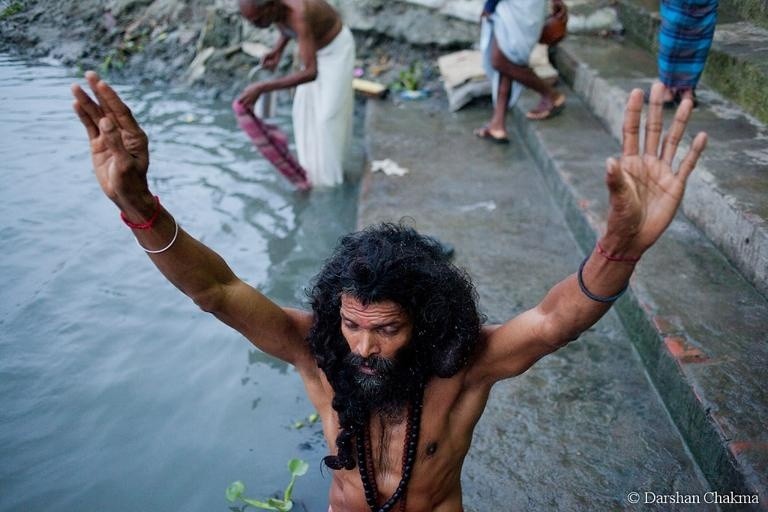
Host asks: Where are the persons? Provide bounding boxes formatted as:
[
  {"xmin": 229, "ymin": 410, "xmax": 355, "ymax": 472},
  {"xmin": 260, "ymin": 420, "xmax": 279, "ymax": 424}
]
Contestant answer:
[
  {"xmin": 643, "ymin": 0, "xmax": 720, "ymax": 114},
  {"xmin": 234, "ymin": 1, "xmax": 357, "ymax": 191},
  {"xmin": 66, "ymin": 65, "xmax": 710, "ymax": 512},
  {"xmin": 471, "ymin": 1, "xmax": 569, "ymax": 145}
]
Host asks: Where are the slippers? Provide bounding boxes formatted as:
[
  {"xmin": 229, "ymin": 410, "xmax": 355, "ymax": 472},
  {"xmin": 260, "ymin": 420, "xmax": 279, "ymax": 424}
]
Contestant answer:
[
  {"xmin": 474, "ymin": 127, "xmax": 509, "ymax": 144},
  {"xmin": 527, "ymin": 97, "xmax": 565, "ymax": 120}
]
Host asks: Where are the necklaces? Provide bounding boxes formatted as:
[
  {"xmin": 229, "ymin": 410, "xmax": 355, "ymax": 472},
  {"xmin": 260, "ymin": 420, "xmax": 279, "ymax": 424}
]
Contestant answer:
[{"xmin": 346, "ymin": 381, "xmax": 424, "ymax": 512}]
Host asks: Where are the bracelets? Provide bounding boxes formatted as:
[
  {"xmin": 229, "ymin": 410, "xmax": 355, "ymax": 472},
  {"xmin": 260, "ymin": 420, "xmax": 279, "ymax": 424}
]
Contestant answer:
[
  {"xmin": 578, "ymin": 257, "xmax": 628, "ymax": 304},
  {"xmin": 127, "ymin": 218, "xmax": 180, "ymax": 255},
  {"xmin": 595, "ymin": 241, "xmax": 640, "ymax": 263},
  {"xmin": 117, "ymin": 193, "xmax": 161, "ymax": 235}
]
